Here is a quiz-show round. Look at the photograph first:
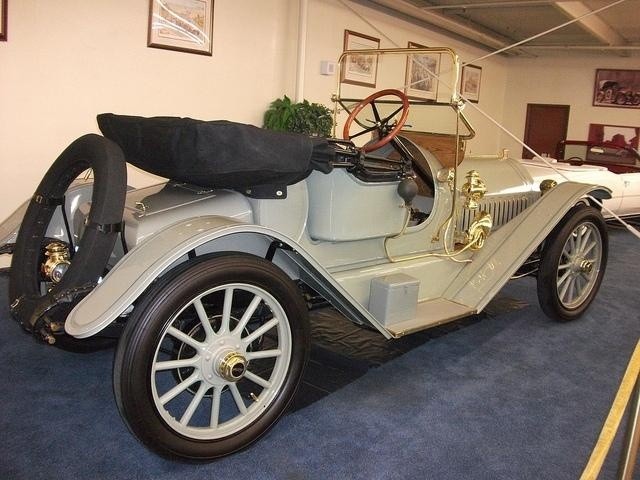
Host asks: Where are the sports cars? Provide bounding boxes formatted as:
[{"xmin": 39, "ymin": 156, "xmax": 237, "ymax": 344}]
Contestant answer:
[{"xmin": 518, "ymin": 139, "xmax": 640, "ymax": 222}]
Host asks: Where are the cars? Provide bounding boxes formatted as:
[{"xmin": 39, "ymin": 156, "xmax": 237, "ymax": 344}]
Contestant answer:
[
  {"xmin": 0, "ymin": 47, "xmax": 612, "ymax": 464},
  {"xmin": 594, "ymin": 79, "xmax": 639, "ymax": 104}
]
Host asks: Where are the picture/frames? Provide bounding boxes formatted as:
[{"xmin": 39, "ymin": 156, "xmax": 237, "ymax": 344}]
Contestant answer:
[
  {"xmin": 592, "ymin": 68, "xmax": 640, "ymax": 109},
  {"xmin": 148, "ymin": 1, "xmax": 214, "ymax": 57},
  {"xmin": 403, "ymin": 40, "xmax": 441, "ymax": 102},
  {"xmin": 460, "ymin": 63, "xmax": 482, "ymax": 104},
  {"xmin": 340, "ymin": 28, "xmax": 380, "ymax": 88}
]
